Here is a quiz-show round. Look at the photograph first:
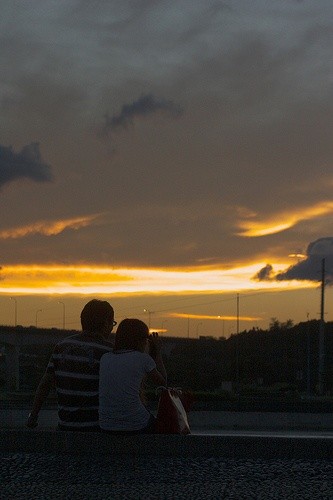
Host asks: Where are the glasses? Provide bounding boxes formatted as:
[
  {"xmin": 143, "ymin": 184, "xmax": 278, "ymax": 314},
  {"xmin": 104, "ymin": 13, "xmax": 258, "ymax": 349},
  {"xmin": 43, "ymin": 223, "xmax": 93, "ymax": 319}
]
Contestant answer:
[
  {"xmin": 108, "ymin": 319, "xmax": 117, "ymax": 327},
  {"xmin": 141, "ymin": 334, "xmax": 152, "ymax": 343}
]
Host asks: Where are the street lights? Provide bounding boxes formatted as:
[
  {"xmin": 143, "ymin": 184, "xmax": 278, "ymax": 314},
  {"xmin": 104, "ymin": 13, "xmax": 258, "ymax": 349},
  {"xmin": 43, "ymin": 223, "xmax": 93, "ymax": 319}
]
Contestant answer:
[
  {"xmin": 307, "ymin": 310, "xmax": 330, "ymax": 397},
  {"xmin": 9, "ymin": 297, "xmax": 17, "ymax": 326},
  {"xmin": 57, "ymin": 300, "xmax": 65, "ymax": 330},
  {"xmin": 218, "ymin": 314, "xmax": 225, "ymax": 336},
  {"xmin": 143, "ymin": 309, "xmax": 151, "ymax": 328},
  {"xmin": 35, "ymin": 310, "xmax": 43, "ymax": 328}
]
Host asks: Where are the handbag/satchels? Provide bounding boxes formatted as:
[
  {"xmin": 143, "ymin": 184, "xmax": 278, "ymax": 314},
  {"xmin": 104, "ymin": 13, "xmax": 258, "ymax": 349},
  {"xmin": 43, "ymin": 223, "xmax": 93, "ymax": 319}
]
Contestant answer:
[{"xmin": 156, "ymin": 389, "xmax": 191, "ymax": 434}]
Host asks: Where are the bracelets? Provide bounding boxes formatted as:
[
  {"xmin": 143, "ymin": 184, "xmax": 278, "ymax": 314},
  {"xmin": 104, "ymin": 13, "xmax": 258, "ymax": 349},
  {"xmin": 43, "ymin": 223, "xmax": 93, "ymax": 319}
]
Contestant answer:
[{"xmin": 26, "ymin": 411, "xmax": 40, "ymax": 420}]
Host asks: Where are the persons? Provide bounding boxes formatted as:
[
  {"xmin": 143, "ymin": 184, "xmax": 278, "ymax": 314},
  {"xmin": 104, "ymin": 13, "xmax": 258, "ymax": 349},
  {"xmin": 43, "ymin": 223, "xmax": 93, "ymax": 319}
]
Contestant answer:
[
  {"xmin": 97, "ymin": 319, "xmax": 183, "ymax": 435},
  {"xmin": 25, "ymin": 299, "xmax": 117, "ymax": 432}
]
